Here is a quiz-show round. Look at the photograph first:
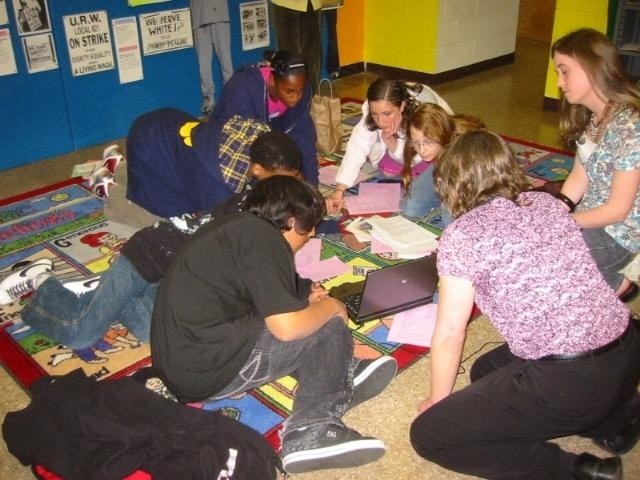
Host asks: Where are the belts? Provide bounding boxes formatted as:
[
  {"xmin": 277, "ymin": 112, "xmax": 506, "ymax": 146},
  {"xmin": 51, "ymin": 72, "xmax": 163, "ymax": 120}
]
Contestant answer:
[{"xmin": 541, "ymin": 331, "xmax": 631, "ymax": 360}]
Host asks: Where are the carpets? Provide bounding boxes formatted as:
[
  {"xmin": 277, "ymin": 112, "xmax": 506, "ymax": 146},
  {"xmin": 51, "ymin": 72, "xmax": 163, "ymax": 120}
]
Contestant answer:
[{"xmin": 1, "ymin": 97, "xmax": 581, "ymax": 456}]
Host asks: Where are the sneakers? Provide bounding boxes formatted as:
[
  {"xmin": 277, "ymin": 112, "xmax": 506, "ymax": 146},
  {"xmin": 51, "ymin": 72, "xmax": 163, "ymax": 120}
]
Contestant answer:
[
  {"xmin": 200, "ymin": 94, "xmax": 215, "ymax": 114},
  {"xmin": 279, "ymin": 420, "xmax": 387, "ymax": 474},
  {"xmin": 103, "ymin": 144, "xmax": 125, "ymax": 174},
  {"xmin": 349, "ymin": 354, "xmax": 398, "ymax": 409},
  {"xmin": 1, "ymin": 257, "xmax": 56, "ymax": 306},
  {"xmin": 89, "ymin": 167, "xmax": 115, "ymax": 197},
  {"xmin": 60, "ymin": 271, "xmax": 103, "ymax": 296}
]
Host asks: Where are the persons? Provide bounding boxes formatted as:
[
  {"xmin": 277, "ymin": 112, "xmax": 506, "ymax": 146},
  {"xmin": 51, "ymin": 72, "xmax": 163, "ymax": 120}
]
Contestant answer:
[
  {"xmin": 320, "ymin": 0, "xmax": 345, "ymax": 80},
  {"xmin": 150, "ymin": 176, "xmax": 398, "ymax": 473},
  {"xmin": 89, "ymin": 108, "xmax": 303, "ymax": 229},
  {"xmin": 551, "ymin": 28, "xmax": 639, "ymax": 297},
  {"xmin": 409, "ymin": 129, "xmax": 639, "ymax": 478},
  {"xmin": 271, "ymin": 0, "xmax": 322, "ymax": 95},
  {"xmin": 0, "ymin": 182, "xmax": 330, "ymax": 351},
  {"xmin": 323, "ymin": 78, "xmax": 455, "ymax": 219},
  {"xmin": 190, "ymin": 1, "xmax": 234, "ymax": 113},
  {"xmin": 212, "ymin": 50, "xmax": 318, "ymax": 196},
  {"xmin": 401, "ymin": 105, "xmax": 488, "ymax": 226}
]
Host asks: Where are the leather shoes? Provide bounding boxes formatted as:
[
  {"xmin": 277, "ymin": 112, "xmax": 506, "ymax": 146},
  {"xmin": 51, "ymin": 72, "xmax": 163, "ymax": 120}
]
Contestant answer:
[
  {"xmin": 594, "ymin": 422, "xmax": 640, "ymax": 453},
  {"xmin": 574, "ymin": 451, "xmax": 622, "ymax": 479}
]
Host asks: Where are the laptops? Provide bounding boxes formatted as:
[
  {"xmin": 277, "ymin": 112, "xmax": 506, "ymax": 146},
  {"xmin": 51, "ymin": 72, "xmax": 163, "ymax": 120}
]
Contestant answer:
[{"xmin": 329, "ymin": 254, "xmax": 439, "ymax": 325}]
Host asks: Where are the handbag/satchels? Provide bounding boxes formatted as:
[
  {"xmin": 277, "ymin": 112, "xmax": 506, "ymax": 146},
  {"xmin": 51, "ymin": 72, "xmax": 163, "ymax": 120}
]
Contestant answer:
[{"xmin": 309, "ymin": 79, "xmax": 341, "ymax": 156}]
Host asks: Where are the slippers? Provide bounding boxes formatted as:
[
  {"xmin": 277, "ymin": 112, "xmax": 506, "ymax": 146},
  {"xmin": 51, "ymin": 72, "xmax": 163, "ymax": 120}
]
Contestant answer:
[{"xmin": 620, "ymin": 279, "xmax": 640, "ymax": 301}]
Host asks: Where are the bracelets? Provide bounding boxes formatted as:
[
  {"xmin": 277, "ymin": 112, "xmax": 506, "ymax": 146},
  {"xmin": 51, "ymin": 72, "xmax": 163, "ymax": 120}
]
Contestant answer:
[
  {"xmin": 557, "ymin": 193, "xmax": 575, "ymax": 212},
  {"xmin": 333, "ymin": 189, "xmax": 344, "ymax": 195}
]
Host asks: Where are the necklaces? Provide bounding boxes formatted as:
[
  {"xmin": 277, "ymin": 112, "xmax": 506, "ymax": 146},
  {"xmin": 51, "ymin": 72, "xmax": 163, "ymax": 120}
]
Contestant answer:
[{"xmin": 590, "ymin": 97, "xmax": 613, "ymax": 142}]
